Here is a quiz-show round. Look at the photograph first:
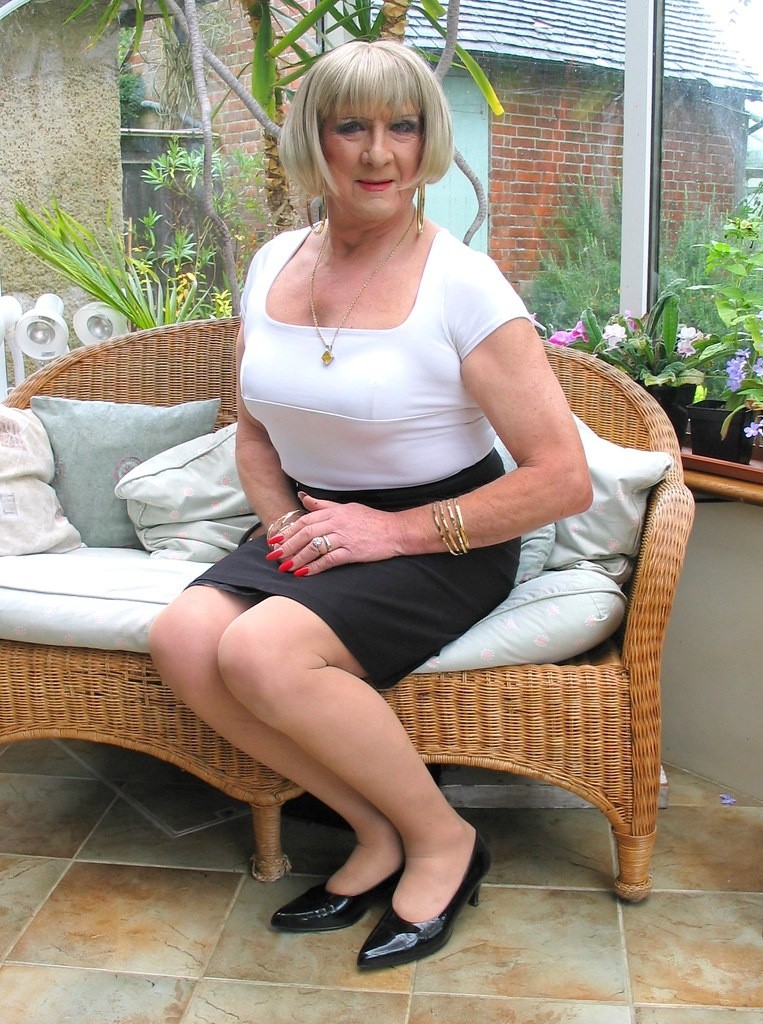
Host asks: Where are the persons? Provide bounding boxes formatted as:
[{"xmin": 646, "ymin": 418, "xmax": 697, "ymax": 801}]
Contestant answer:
[{"xmin": 150, "ymin": 38, "xmax": 593, "ymax": 973}]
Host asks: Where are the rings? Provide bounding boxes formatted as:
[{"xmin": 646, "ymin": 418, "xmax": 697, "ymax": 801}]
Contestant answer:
[
  {"xmin": 312, "ymin": 537, "xmax": 322, "ymax": 556},
  {"xmin": 323, "ymin": 535, "xmax": 332, "ymax": 553}
]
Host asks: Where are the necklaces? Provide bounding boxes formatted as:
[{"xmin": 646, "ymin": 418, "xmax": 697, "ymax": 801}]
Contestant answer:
[{"xmin": 310, "ymin": 203, "xmax": 416, "ymax": 366}]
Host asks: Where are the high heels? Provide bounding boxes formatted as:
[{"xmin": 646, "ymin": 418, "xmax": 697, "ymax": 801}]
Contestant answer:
[
  {"xmin": 271, "ymin": 859, "xmax": 406, "ymax": 932},
  {"xmin": 357, "ymin": 829, "xmax": 492, "ymax": 967}
]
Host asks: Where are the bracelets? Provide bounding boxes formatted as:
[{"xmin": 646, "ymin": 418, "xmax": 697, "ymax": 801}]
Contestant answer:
[
  {"xmin": 266, "ymin": 509, "xmax": 308, "ymax": 548},
  {"xmin": 432, "ymin": 498, "xmax": 472, "ymax": 556}
]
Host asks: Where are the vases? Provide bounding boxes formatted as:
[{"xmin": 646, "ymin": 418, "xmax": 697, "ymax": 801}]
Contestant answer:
[
  {"xmin": 685, "ymin": 398, "xmax": 753, "ymax": 463},
  {"xmin": 635, "ymin": 381, "xmax": 697, "ymax": 451}
]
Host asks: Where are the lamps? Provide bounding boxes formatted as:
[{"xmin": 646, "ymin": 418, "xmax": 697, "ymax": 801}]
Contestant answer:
[{"xmin": 1, "ymin": 289, "xmax": 134, "ymax": 392}]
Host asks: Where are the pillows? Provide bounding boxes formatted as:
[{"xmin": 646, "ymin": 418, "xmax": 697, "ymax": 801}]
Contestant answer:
[
  {"xmin": 115, "ymin": 421, "xmax": 258, "ymax": 563},
  {"xmin": 29, "ymin": 394, "xmax": 222, "ymax": 549},
  {"xmin": 544, "ymin": 409, "xmax": 673, "ymax": 585},
  {"xmin": 0, "ymin": 405, "xmax": 87, "ymax": 562}
]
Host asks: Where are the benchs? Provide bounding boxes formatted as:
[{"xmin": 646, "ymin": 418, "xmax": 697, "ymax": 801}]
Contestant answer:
[{"xmin": 0, "ymin": 318, "xmax": 695, "ymax": 903}]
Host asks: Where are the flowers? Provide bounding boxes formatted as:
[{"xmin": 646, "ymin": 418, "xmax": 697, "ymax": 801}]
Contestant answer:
[{"xmin": 546, "ymin": 278, "xmax": 763, "ymax": 446}]
